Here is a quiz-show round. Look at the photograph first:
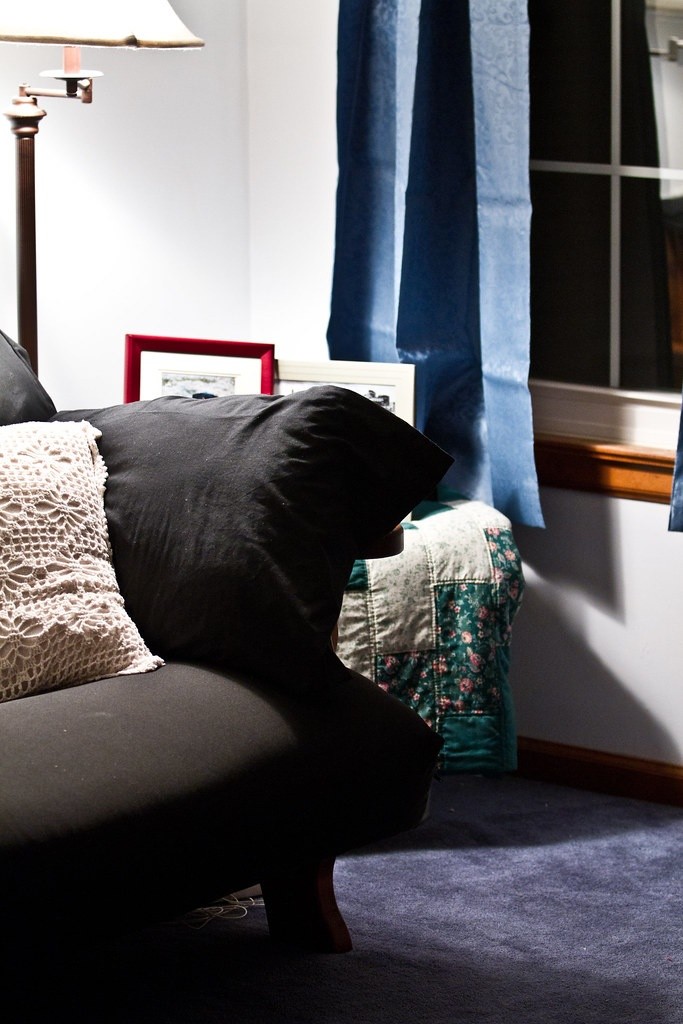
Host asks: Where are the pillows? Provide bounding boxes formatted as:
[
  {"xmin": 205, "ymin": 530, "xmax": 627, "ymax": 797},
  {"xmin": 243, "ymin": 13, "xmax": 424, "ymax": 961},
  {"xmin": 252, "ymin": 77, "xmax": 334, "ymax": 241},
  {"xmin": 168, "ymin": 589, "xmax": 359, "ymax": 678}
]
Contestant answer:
[{"xmin": 0, "ymin": 418, "xmax": 166, "ymax": 704}]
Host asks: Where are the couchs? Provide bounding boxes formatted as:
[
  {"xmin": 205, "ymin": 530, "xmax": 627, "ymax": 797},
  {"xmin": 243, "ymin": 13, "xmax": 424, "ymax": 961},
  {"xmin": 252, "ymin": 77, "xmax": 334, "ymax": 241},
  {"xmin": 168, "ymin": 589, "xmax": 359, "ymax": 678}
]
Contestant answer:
[{"xmin": 0, "ymin": 327, "xmax": 454, "ymax": 955}]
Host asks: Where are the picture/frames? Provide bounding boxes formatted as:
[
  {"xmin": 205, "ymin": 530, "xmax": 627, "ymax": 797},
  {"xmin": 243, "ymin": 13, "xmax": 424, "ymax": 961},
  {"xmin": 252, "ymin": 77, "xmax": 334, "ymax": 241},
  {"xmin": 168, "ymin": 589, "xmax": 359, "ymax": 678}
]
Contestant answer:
[
  {"xmin": 123, "ymin": 333, "xmax": 276, "ymax": 407},
  {"xmin": 274, "ymin": 359, "xmax": 416, "ymax": 426}
]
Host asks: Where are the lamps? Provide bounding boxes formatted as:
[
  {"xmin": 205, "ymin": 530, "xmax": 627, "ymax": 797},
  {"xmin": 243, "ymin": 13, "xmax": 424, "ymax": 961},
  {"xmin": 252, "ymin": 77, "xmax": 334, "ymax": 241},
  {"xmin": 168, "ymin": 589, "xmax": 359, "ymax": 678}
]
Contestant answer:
[{"xmin": 0, "ymin": 0, "xmax": 204, "ymax": 373}]
